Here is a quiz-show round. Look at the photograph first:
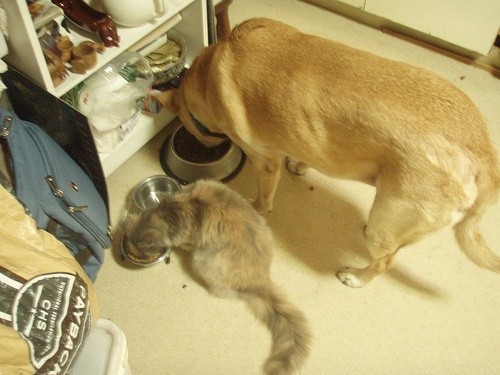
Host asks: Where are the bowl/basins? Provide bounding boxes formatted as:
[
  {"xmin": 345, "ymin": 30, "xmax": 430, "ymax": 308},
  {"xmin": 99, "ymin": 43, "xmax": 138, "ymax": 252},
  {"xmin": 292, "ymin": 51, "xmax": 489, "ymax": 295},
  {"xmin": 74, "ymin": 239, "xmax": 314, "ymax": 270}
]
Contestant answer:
[
  {"xmin": 120, "ymin": 231, "xmax": 171, "ymax": 268},
  {"xmin": 132, "ymin": 174, "xmax": 183, "ymax": 212},
  {"xmin": 160, "ymin": 123, "xmax": 244, "ymax": 185}
]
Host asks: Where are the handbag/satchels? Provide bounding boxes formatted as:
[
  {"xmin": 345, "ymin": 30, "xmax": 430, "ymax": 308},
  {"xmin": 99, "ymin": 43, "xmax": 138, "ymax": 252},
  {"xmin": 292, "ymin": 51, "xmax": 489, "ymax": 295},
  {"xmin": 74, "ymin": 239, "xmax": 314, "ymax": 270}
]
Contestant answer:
[
  {"xmin": 1, "ymin": 183, "xmax": 99, "ymax": 374},
  {"xmin": 0, "ymin": 105, "xmax": 113, "ymax": 283}
]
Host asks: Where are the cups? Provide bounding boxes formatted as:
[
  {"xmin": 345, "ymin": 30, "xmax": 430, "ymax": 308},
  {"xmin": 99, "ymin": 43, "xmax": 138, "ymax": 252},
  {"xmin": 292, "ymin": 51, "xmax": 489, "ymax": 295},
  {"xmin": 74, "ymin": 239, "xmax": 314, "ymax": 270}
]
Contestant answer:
[
  {"xmin": 73, "ymin": 42, "xmax": 96, "ymax": 69},
  {"xmin": 56, "ymin": 35, "xmax": 74, "ymax": 60},
  {"xmin": 101, "ymin": 0, "xmax": 165, "ymax": 27}
]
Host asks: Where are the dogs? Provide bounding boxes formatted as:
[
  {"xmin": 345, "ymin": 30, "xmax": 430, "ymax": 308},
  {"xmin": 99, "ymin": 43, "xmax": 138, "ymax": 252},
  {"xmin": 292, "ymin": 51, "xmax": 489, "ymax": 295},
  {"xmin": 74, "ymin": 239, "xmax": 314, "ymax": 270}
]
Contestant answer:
[{"xmin": 151, "ymin": 17, "xmax": 500, "ymax": 290}]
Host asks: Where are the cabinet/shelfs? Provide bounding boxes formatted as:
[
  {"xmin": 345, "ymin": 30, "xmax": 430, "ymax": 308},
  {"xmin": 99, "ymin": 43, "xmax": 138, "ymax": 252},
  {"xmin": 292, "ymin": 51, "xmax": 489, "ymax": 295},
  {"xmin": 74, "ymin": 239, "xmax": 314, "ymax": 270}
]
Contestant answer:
[{"xmin": 4, "ymin": 0, "xmax": 209, "ymax": 176}]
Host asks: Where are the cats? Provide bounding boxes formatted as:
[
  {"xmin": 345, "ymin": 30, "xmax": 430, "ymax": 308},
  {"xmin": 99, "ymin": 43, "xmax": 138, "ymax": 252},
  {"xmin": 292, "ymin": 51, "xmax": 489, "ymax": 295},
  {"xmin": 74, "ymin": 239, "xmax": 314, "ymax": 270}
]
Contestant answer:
[{"xmin": 118, "ymin": 179, "xmax": 314, "ymax": 375}]
